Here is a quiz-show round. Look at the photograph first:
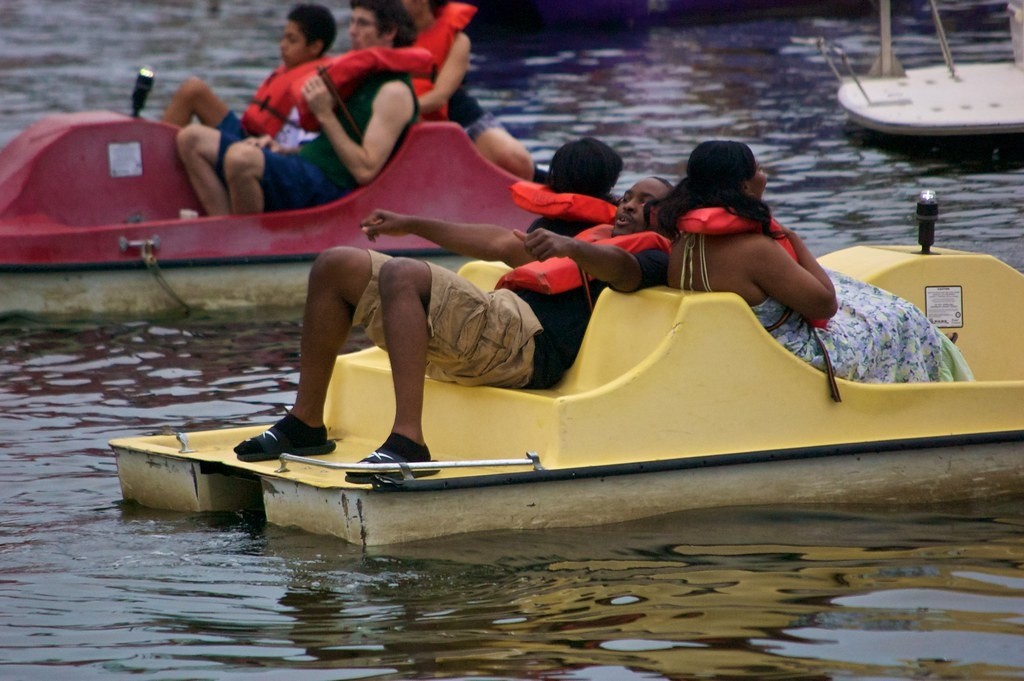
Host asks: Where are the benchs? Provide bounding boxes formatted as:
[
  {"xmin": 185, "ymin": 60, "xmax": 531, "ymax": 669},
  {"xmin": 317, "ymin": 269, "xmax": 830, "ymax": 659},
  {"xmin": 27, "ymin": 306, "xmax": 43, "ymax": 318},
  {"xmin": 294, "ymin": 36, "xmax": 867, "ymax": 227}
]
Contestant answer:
[
  {"xmin": 320, "ymin": 239, "xmax": 1024, "ymax": 470},
  {"xmin": 183, "ymin": 120, "xmax": 547, "ymax": 259}
]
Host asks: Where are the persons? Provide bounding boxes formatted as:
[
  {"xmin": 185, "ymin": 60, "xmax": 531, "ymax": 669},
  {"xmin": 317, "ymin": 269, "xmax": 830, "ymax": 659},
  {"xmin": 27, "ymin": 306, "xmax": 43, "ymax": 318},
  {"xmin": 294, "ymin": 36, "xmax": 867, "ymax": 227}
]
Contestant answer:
[
  {"xmin": 161, "ymin": 3, "xmax": 338, "ymax": 140},
  {"xmin": 177, "ymin": 0, "xmax": 419, "ymax": 216},
  {"xmin": 668, "ymin": 140, "xmax": 973, "ymax": 384},
  {"xmin": 233, "ymin": 177, "xmax": 674, "ymax": 484},
  {"xmin": 401, "ymin": 0, "xmax": 547, "ymax": 184}
]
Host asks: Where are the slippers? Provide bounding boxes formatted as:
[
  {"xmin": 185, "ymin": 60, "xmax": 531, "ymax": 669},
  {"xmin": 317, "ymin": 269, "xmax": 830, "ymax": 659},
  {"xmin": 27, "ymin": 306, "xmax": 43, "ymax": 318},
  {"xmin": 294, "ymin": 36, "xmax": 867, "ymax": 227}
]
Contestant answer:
[
  {"xmin": 344, "ymin": 451, "xmax": 441, "ymax": 485},
  {"xmin": 236, "ymin": 428, "xmax": 338, "ymax": 463}
]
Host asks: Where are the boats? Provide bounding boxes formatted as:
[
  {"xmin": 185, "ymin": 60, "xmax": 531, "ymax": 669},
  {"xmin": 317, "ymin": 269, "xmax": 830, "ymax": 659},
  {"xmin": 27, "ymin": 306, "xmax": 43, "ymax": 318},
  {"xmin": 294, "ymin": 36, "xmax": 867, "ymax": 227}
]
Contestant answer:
[
  {"xmin": 814, "ymin": 1, "xmax": 1024, "ymax": 141},
  {"xmin": 1, "ymin": 1, "xmax": 567, "ymax": 267},
  {"xmin": 110, "ymin": 194, "xmax": 1023, "ymax": 546}
]
